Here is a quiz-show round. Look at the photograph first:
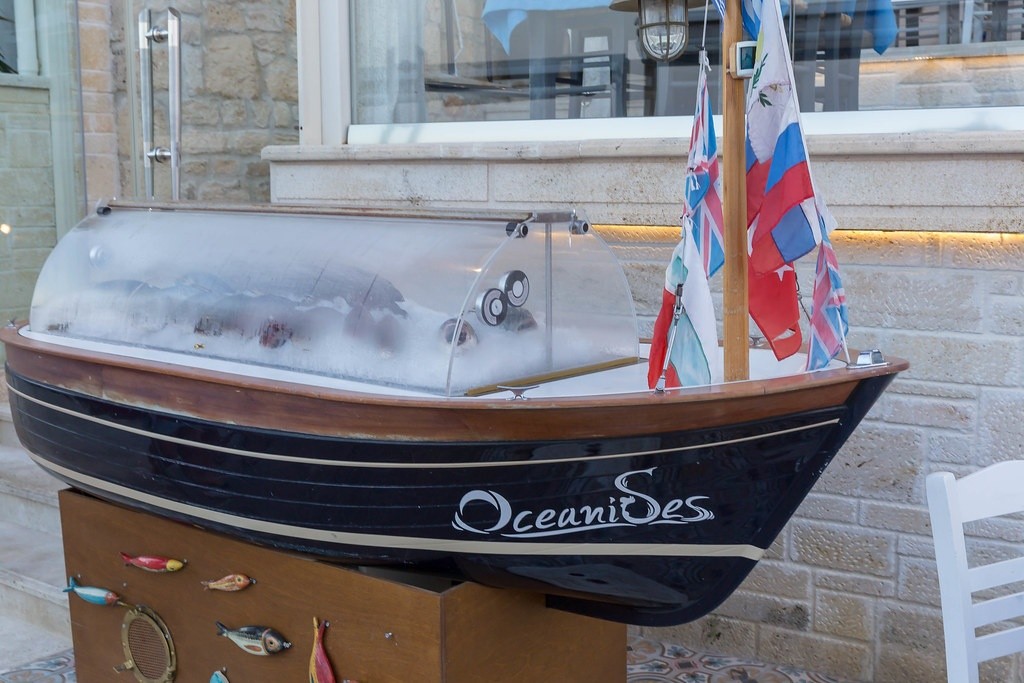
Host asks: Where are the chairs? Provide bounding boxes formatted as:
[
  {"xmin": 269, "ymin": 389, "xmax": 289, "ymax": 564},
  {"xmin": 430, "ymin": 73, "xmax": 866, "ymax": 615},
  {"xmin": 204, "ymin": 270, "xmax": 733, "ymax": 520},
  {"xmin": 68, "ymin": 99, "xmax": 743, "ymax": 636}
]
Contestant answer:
[
  {"xmin": 925, "ymin": 459, "xmax": 1024, "ymax": 683},
  {"xmin": 553, "ymin": 0, "xmax": 868, "ymax": 119}
]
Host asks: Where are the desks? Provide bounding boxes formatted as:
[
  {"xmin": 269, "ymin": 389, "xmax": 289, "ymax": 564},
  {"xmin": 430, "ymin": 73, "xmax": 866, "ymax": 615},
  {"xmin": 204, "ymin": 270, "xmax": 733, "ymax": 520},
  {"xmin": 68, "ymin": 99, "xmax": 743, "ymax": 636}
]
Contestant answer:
[{"xmin": 481, "ymin": 0, "xmax": 899, "ymax": 120}]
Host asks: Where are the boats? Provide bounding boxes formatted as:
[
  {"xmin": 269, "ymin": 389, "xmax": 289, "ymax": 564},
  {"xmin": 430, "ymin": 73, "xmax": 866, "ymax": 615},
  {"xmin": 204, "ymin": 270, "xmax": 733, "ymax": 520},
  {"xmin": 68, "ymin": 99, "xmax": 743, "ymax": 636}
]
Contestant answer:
[{"xmin": 2, "ymin": 2, "xmax": 908, "ymax": 629}]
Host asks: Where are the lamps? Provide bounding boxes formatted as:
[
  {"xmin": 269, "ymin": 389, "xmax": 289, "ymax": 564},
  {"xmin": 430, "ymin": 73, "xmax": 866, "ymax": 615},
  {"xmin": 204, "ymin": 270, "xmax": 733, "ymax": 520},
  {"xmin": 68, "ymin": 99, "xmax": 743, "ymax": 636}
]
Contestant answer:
[{"xmin": 609, "ymin": 0, "xmax": 713, "ymax": 61}]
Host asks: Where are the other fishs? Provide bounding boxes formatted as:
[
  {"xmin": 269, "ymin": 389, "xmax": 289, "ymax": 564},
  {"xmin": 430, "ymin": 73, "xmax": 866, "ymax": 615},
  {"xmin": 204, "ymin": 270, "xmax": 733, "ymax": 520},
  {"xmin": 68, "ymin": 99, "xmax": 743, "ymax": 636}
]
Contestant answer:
[
  {"xmin": 216, "ymin": 622, "xmax": 292, "ymax": 656},
  {"xmin": 209, "ymin": 670, "xmax": 229, "ymax": 683},
  {"xmin": 308, "ymin": 617, "xmax": 336, "ymax": 683},
  {"xmin": 118, "ymin": 551, "xmax": 184, "ymax": 573},
  {"xmin": 63, "ymin": 576, "xmax": 119, "ymax": 606},
  {"xmin": 200, "ymin": 573, "xmax": 255, "ymax": 592}
]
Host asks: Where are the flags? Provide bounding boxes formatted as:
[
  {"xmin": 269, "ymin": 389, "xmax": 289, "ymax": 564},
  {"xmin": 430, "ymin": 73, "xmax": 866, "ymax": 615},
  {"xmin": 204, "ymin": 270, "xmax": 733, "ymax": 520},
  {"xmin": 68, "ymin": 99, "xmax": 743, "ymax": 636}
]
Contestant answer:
[{"xmin": 648, "ymin": 0, "xmax": 848, "ymax": 390}]
